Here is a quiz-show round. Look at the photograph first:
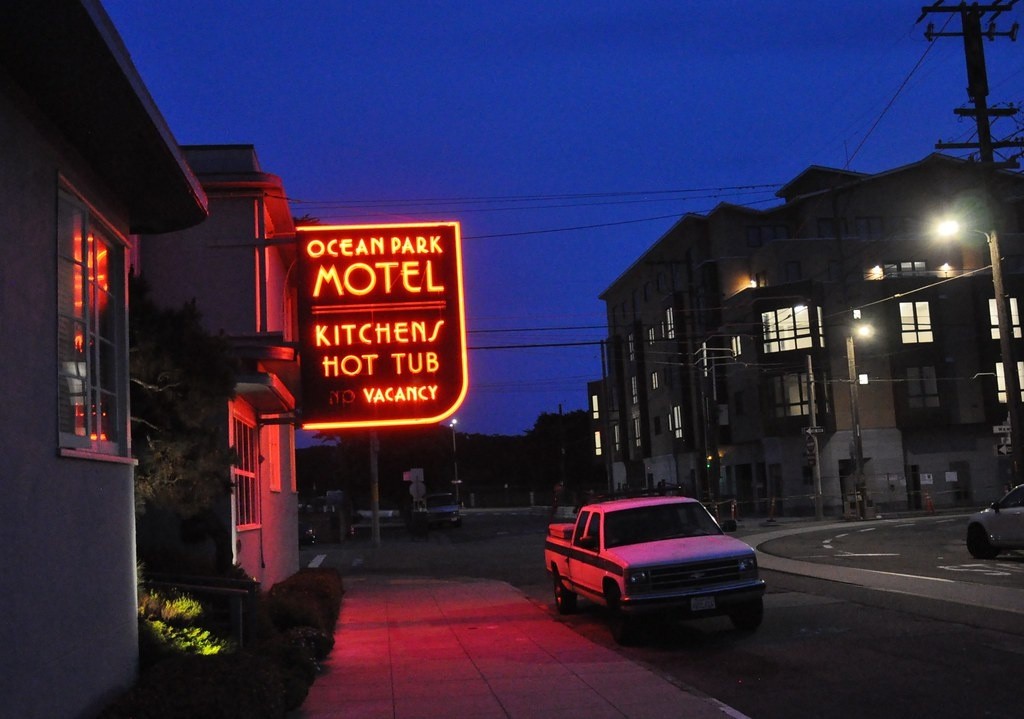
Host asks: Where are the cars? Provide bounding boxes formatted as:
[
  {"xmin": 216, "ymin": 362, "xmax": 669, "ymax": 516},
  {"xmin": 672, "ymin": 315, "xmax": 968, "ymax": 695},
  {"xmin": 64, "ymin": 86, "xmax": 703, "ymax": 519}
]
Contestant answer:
[
  {"xmin": 966, "ymin": 484, "xmax": 1024, "ymax": 561},
  {"xmin": 424, "ymin": 492, "xmax": 463, "ymax": 527}
]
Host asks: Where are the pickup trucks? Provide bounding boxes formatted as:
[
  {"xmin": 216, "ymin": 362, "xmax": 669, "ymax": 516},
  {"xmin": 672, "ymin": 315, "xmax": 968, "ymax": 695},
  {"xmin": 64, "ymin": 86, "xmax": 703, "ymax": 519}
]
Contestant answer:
[{"xmin": 545, "ymin": 495, "xmax": 767, "ymax": 648}]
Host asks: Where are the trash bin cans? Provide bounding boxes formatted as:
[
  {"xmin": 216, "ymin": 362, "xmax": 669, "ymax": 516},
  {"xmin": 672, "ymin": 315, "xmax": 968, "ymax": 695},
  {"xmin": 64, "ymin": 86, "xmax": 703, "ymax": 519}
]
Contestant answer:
[{"xmin": 411, "ymin": 509, "xmax": 429, "ymax": 538}]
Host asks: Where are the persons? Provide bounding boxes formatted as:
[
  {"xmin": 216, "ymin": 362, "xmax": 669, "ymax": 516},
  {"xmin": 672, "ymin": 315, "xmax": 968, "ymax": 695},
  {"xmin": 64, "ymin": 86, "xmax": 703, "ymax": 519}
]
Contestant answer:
[{"xmin": 1003, "ymin": 480, "xmax": 1016, "ymax": 494}]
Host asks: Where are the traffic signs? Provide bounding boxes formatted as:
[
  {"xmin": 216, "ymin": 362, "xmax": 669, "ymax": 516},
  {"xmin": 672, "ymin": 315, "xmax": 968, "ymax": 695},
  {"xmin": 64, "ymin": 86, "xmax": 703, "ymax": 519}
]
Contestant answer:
[{"xmin": 804, "ymin": 426, "xmax": 824, "ymax": 435}]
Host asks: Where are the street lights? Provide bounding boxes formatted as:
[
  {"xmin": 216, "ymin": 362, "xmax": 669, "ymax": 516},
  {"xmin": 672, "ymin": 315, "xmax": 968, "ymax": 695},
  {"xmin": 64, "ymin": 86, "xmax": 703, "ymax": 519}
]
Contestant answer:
[
  {"xmin": 449, "ymin": 419, "xmax": 461, "ymax": 504},
  {"xmin": 933, "ymin": 218, "xmax": 1024, "ymax": 488},
  {"xmin": 845, "ymin": 321, "xmax": 876, "ymax": 518}
]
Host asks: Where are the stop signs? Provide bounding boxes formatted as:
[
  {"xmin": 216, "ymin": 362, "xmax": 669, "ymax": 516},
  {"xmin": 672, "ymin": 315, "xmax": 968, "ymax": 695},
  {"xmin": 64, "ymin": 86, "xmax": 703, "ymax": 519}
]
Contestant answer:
[{"xmin": 807, "ymin": 436, "xmax": 816, "ymax": 453}]
